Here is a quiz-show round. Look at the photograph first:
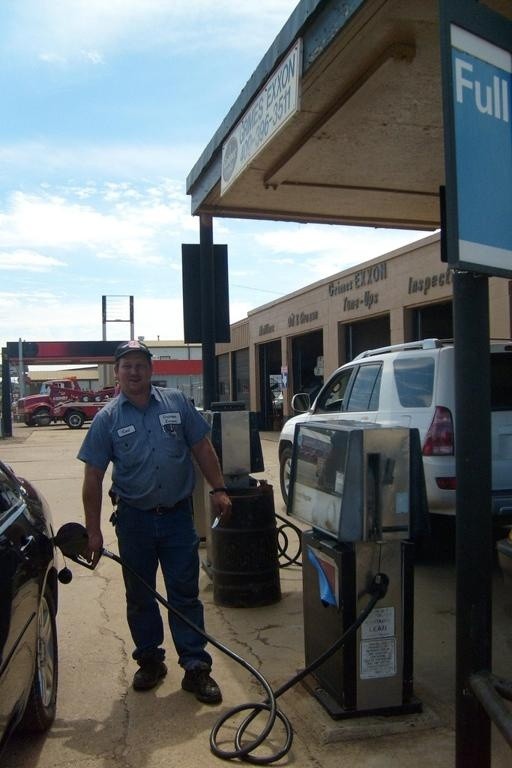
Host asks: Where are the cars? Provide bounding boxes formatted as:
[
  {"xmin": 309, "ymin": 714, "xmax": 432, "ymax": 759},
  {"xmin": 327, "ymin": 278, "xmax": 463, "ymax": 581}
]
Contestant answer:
[{"xmin": 0, "ymin": 461, "xmax": 72, "ymax": 756}]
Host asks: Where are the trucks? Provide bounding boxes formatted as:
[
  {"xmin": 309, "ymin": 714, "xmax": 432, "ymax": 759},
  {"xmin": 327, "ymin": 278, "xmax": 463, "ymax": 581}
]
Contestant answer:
[{"xmin": 11, "ymin": 377, "xmax": 121, "ymax": 429}]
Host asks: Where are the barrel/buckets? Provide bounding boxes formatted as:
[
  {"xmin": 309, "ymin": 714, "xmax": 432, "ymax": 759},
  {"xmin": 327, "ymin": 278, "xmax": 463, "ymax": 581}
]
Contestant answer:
[{"xmin": 209, "ymin": 485, "xmax": 282, "ymax": 608}]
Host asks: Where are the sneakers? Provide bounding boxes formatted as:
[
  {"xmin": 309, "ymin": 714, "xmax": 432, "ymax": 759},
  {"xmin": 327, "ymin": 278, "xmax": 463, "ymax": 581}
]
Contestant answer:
[
  {"xmin": 182, "ymin": 669, "xmax": 223, "ymax": 703},
  {"xmin": 133, "ymin": 662, "xmax": 168, "ymax": 690}
]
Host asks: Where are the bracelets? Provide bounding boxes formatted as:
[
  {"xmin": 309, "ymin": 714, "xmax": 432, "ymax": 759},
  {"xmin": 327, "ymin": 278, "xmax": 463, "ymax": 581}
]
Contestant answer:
[{"xmin": 212, "ymin": 488, "xmax": 228, "ymax": 492}]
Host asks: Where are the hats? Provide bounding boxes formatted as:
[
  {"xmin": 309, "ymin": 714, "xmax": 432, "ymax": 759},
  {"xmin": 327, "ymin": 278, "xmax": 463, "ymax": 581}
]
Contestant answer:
[{"xmin": 115, "ymin": 340, "xmax": 152, "ymax": 360}]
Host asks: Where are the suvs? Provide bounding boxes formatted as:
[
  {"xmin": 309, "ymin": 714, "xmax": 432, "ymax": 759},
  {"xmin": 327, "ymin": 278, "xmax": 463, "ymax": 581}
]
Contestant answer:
[{"xmin": 278, "ymin": 337, "xmax": 511, "ymax": 528}]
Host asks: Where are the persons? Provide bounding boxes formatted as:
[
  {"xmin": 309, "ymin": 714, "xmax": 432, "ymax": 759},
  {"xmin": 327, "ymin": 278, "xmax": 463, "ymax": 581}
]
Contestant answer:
[{"xmin": 77, "ymin": 343, "xmax": 232, "ymax": 704}]
